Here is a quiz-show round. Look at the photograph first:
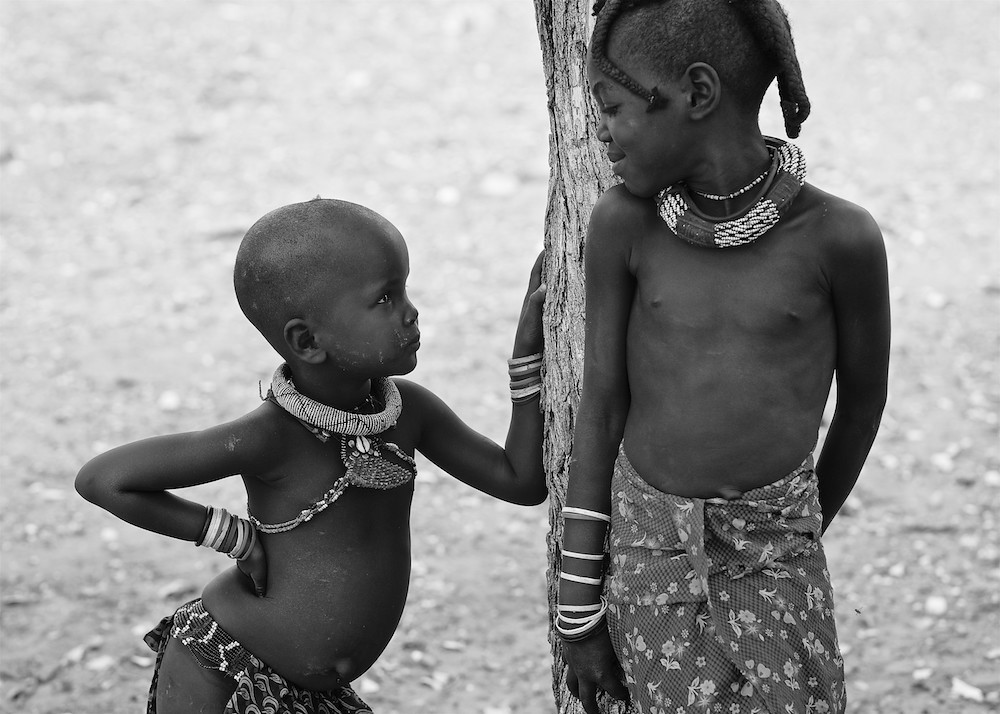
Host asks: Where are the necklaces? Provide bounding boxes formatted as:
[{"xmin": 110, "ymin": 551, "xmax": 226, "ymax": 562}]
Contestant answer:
[
  {"xmin": 271, "ymin": 363, "xmax": 403, "ymax": 434},
  {"xmin": 656, "ymin": 135, "xmax": 806, "ymax": 250}
]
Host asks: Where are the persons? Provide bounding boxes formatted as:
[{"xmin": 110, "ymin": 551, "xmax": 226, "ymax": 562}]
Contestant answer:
[
  {"xmin": 74, "ymin": 199, "xmax": 546, "ymax": 714},
  {"xmin": 554, "ymin": 0, "xmax": 891, "ymax": 714}
]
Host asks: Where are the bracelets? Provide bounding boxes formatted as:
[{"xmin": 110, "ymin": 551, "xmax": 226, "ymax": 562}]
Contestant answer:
[
  {"xmin": 555, "ymin": 507, "xmax": 608, "ymax": 644},
  {"xmin": 195, "ymin": 504, "xmax": 256, "ymax": 562},
  {"xmin": 507, "ymin": 354, "xmax": 542, "ymax": 406}
]
